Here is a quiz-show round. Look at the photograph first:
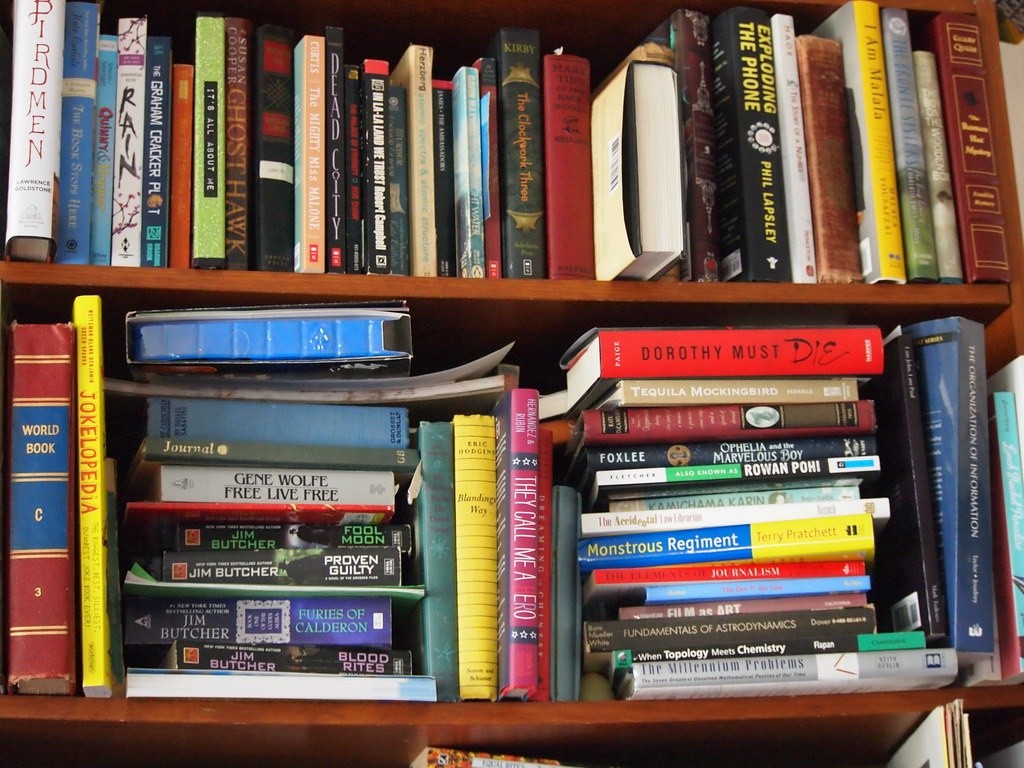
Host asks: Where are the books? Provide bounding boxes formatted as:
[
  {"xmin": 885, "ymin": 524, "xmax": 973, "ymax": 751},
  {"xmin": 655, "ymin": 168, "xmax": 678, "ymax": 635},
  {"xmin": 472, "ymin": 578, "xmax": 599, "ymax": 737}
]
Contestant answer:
[{"xmin": 0, "ymin": 0, "xmax": 1024, "ymax": 768}]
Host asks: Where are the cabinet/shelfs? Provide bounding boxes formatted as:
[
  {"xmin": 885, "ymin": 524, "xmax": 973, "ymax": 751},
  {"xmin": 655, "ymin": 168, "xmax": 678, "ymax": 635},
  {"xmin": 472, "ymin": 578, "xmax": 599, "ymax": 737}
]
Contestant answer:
[{"xmin": 0, "ymin": 260, "xmax": 1024, "ymax": 768}]
